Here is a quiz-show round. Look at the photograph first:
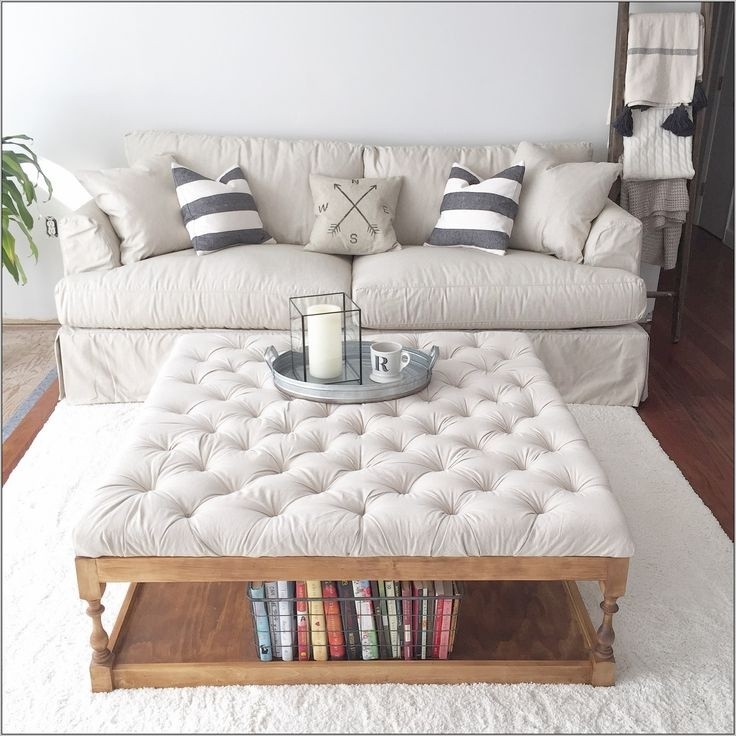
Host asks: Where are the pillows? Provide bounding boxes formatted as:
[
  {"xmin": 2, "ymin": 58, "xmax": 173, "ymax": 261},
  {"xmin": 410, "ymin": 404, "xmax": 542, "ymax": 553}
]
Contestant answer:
[
  {"xmin": 74, "ymin": 154, "xmax": 194, "ymax": 265},
  {"xmin": 510, "ymin": 140, "xmax": 623, "ymax": 264},
  {"xmin": 304, "ymin": 172, "xmax": 403, "ymax": 255},
  {"xmin": 423, "ymin": 161, "xmax": 525, "ymax": 255},
  {"xmin": 169, "ymin": 155, "xmax": 278, "ymax": 258}
]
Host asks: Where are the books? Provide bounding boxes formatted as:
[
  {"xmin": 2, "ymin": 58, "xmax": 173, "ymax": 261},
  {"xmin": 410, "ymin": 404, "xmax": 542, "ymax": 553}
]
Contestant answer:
[{"xmin": 249, "ymin": 580, "xmax": 461, "ymax": 662}]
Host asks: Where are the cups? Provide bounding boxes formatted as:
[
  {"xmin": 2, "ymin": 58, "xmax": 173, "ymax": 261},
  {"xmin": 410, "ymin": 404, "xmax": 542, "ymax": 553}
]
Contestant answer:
[{"xmin": 368, "ymin": 341, "xmax": 410, "ymax": 383}]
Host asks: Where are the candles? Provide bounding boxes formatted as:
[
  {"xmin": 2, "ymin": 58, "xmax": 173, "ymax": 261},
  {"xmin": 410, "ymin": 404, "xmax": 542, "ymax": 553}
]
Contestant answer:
[{"xmin": 307, "ymin": 303, "xmax": 344, "ymax": 379}]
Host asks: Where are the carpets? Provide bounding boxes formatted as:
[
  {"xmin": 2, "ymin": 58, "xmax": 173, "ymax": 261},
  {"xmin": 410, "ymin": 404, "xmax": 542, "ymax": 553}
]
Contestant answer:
[{"xmin": 0, "ymin": 398, "xmax": 735, "ymax": 735}]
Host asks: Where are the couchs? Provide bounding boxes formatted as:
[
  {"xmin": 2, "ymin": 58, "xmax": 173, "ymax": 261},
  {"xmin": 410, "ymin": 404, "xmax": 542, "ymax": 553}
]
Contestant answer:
[{"xmin": 56, "ymin": 129, "xmax": 651, "ymax": 408}]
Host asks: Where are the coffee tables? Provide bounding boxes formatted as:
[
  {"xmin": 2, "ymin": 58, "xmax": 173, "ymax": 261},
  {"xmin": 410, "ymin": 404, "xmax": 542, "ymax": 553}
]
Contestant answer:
[{"xmin": 76, "ymin": 334, "xmax": 636, "ymax": 693}]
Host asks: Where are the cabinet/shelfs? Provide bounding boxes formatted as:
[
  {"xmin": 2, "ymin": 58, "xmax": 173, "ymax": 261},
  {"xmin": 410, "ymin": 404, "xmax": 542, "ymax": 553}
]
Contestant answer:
[{"xmin": 606, "ymin": 3, "xmax": 720, "ymax": 344}]
